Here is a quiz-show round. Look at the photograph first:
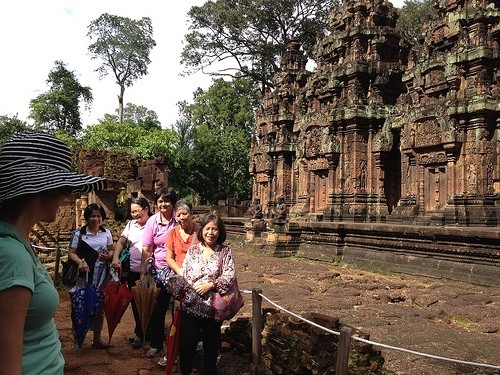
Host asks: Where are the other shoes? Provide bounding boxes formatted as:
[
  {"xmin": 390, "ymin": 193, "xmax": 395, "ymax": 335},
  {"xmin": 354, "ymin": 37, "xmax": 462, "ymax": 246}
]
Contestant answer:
[
  {"xmin": 132, "ymin": 340, "xmax": 143, "ymax": 349},
  {"xmin": 146, "ymin": 346, "xmax": 166, "ymax": 357}
]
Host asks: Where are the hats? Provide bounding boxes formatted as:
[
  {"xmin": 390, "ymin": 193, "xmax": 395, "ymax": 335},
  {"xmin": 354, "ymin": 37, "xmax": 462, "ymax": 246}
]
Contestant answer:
[{"xmin": 0, "ymin": 131, "xmax": 109, "ymax": 203}]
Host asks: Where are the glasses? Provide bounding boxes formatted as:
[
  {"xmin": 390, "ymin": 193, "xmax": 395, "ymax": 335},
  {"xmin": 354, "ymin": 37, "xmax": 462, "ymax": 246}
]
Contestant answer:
[
  {"xmin": 129, "ymin": 207, "xmax": 146, "ymax": 215},
  {"xmin": 35, "ymin": 186, "xmax": 74, "ymax": 199}
]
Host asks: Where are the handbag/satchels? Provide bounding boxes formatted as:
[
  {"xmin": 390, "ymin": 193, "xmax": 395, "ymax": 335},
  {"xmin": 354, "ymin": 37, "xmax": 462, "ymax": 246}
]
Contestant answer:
[
  {"xmin": 60, "ymin": 229, "xmax": 82, "ymax": 286},
  {"xmin": 144, "ymin": 264, "xmax": 165, "ymax": 293},
  {"xmin": 115, "ymin": 220, "xmax": 130, "ymax": 284},
  {"xmin": 212, "ymin": 246, "xmax": 244, "ymax": 323}
]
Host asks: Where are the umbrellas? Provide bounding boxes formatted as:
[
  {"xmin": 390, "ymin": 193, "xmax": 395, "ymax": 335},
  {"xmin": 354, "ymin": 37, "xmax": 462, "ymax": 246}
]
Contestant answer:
[
  {"xmin": 131, "ymin": 257, "xmax": 161, "ymax": 344},
  {"xmin": 69, "ymin": 267, "xmax": 106, "ymax": 356},
  {"xmin": 102, "ymin": 264, "xmax": 134, "ymax": 345},
  {"xmin": 166, "ymin": 307, "xmax": 182, "ymax": 375}
]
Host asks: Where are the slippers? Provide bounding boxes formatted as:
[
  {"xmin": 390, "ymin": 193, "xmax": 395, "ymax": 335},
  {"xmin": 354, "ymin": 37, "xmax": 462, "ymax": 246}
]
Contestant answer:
[{"xmin": 91, "ymin": 339, "xmax": 113, "ymax": 348}]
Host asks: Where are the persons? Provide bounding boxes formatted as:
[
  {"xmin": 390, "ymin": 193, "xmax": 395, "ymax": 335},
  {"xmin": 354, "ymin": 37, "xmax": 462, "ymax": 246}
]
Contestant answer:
[
  {"xmin": 113, "ymin": 187, "xmax": 236, "ymax": 375},
  {"xmin": 270, "ymin": 194, "xmax": 288, "ymax": 219},
  {"xmin": 69, "ymin": 202, "xmax": 115, "ymax": 349},
  {"xmin": 249, "ymin": 196, "xmax": 263, "ymax": 219},
  {"xmin": 0, "ymin": 128, "xmax": 110, "ymax": 375}
]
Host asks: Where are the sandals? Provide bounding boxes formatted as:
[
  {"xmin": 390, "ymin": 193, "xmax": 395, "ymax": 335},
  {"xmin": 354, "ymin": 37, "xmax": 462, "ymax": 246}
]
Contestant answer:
[{"xmin": 157, "ymin": 355, "xmax": 167, "ymax": 366}]
